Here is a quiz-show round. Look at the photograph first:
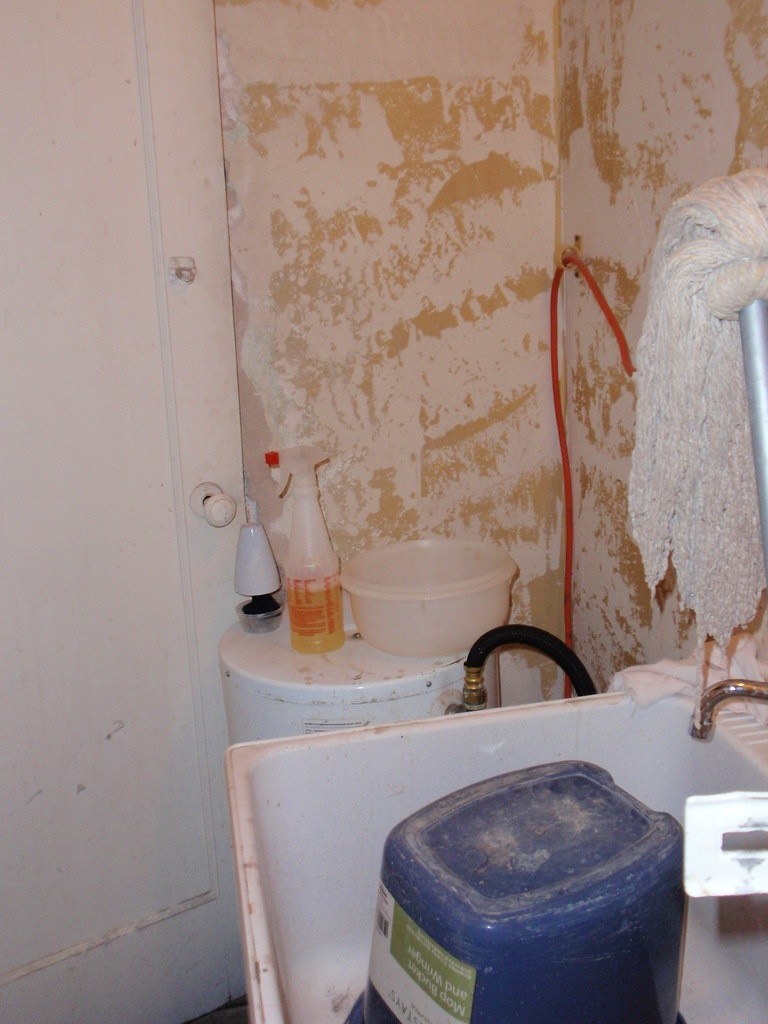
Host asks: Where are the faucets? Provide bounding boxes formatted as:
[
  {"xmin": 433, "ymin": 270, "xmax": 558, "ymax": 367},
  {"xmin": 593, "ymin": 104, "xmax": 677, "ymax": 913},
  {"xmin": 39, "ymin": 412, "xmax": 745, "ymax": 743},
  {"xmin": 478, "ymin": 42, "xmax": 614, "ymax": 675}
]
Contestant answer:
[{"xmin": 687, "ymin": 678, "xmax": 768, "ymax": 743}]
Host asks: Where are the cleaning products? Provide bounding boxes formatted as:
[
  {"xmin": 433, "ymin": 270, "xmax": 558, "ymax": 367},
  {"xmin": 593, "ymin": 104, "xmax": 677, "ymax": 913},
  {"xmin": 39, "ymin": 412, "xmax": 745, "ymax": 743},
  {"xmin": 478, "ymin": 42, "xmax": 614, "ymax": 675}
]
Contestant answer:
[{"xmin": 263, "ymin": 443, "xmax": 346, "ymax": 654}]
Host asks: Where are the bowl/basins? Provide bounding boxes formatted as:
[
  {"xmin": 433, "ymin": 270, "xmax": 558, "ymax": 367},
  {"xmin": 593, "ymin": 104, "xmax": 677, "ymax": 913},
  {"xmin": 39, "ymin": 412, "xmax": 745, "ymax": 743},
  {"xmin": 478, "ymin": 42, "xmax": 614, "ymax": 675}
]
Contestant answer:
[{"xmin": 335, "ymin": 539, "xmax": 518, "ymax": 655}]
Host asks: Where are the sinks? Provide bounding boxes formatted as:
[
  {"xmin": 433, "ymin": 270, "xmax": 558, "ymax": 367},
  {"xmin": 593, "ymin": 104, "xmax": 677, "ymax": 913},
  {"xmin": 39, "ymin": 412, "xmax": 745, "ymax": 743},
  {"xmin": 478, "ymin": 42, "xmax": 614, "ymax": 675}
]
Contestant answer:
[{"xmin": 221, "ymin": 686, "xmax": 768, "ymax": 1024}]
